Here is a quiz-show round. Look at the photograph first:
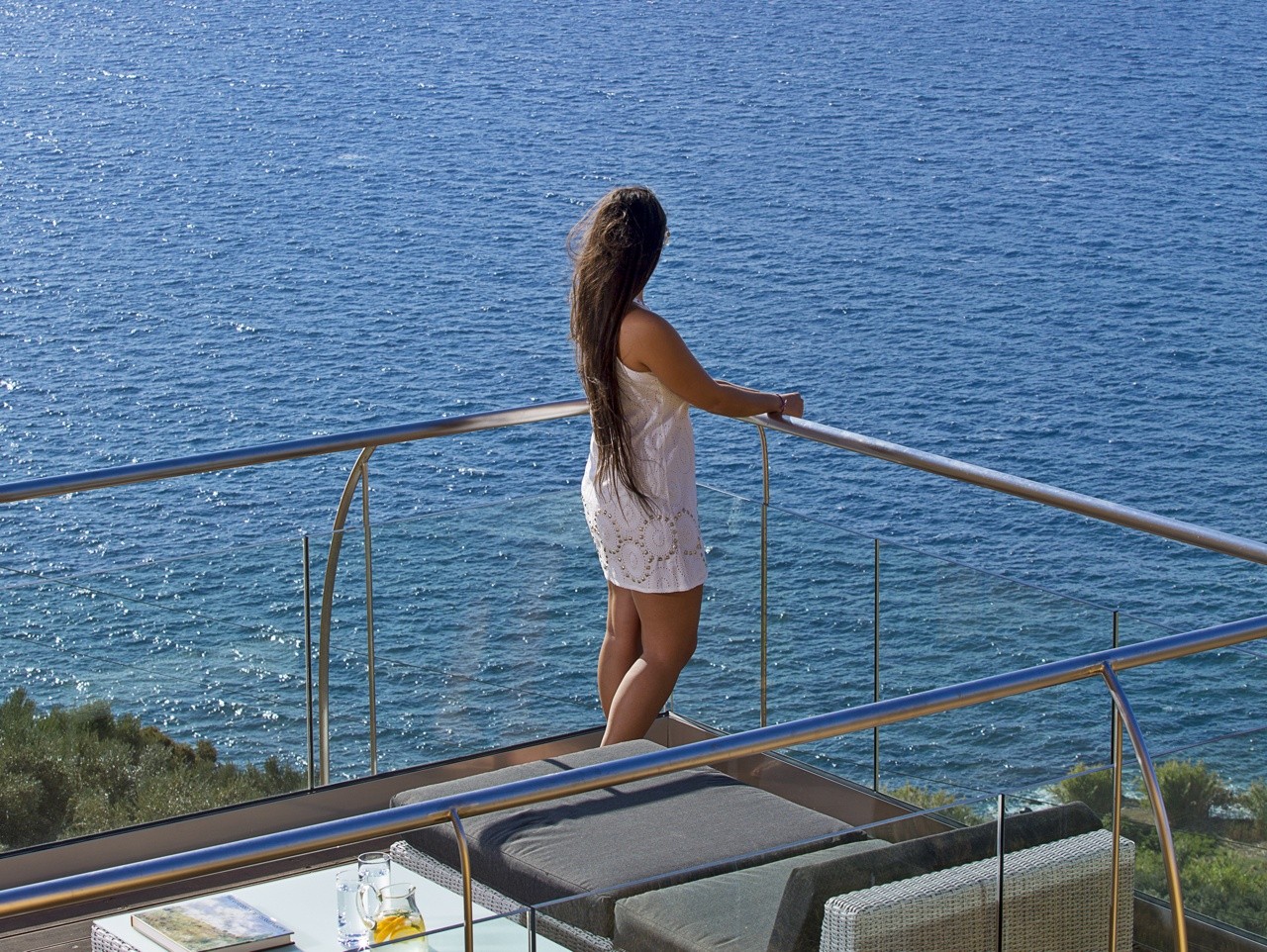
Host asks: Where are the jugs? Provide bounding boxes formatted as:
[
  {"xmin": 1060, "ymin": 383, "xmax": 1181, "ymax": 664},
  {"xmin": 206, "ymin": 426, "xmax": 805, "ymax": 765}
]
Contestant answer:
[{"xmin": 356, "ymin": 882, "xmax": 428, "ymax": 952}]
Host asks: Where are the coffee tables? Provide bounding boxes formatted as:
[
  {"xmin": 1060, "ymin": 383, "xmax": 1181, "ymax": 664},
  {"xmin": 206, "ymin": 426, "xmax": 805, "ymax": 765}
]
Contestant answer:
[{"xmin": 88, "ymin": 855, "xmax": 573, "ymax": 952}]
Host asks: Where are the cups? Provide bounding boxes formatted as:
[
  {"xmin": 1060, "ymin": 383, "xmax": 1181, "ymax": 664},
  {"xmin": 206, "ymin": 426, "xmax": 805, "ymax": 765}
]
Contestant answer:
[
  {"xmin": 336, "ymin": 869, "xmax": 371, "ymax": 952},
  {"xmin": 355, "ymin": 851, "xmax": 392, "ymax": 914}
]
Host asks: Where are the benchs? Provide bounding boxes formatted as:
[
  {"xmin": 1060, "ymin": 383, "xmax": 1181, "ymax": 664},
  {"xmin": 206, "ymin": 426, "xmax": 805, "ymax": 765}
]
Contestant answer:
[{"xmin": 390, "ymin": 738, "xmax": 1103, "ymax": 952}]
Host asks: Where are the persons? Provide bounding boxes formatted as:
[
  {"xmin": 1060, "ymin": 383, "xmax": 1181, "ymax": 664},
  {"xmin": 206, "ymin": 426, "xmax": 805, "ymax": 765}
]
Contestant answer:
[{"xmin": 565, "ymin": 181, "xmax": 805, "ymax": 747}]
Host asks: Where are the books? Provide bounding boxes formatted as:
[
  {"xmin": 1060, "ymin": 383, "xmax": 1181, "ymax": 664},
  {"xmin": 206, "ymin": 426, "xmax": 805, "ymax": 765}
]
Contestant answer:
[{"xmin": 131, "ymin": 894, "xmax": 295, "ymax": 951}]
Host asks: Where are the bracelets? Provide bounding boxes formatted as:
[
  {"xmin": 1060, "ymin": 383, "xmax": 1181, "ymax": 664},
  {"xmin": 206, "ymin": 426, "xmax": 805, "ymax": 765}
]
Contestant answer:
[{"xmin": 776, "ymin": 393, "xmax": 786, "ymax": 417}]
